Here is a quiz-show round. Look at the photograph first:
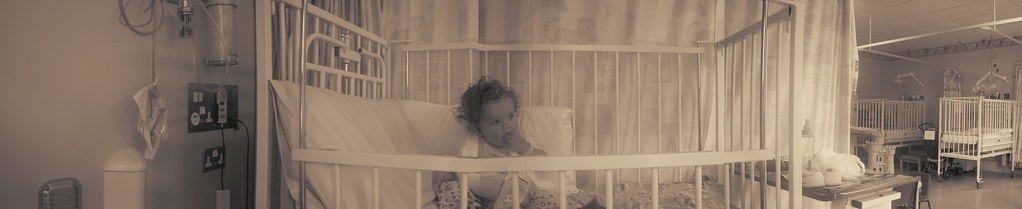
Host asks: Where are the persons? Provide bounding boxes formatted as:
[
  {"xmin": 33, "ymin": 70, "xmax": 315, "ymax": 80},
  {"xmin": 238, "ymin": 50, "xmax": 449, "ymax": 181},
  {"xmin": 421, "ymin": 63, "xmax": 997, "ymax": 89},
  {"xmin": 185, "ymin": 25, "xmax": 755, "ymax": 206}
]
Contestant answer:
[{"xmin": 437, "ymin": 77, "xmax": 559, "ymax": 209}]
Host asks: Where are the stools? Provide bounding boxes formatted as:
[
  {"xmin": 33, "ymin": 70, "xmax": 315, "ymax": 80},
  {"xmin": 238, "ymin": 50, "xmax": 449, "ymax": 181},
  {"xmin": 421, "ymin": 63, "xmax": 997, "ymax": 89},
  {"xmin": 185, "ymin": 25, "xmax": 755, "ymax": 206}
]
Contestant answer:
[{"xmin": 899, "ymin": 153, "xmax": 928, "ymax": 172}]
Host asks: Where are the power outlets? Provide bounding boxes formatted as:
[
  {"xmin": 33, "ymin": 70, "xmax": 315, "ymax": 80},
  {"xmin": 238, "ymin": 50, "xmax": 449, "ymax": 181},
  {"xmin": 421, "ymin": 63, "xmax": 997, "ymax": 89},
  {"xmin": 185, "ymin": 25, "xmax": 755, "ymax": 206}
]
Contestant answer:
[{"xmin": 202, "ymin": 144, "xmax": 227, "ymax": 173}]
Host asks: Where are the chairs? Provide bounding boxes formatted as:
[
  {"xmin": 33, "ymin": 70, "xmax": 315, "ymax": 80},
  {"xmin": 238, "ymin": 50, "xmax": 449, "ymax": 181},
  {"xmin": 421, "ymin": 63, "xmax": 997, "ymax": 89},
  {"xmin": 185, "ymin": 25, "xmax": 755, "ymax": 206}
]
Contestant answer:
[{"xmin": 910, "ymin": 171, "xmax": 931, "ymax": 209}]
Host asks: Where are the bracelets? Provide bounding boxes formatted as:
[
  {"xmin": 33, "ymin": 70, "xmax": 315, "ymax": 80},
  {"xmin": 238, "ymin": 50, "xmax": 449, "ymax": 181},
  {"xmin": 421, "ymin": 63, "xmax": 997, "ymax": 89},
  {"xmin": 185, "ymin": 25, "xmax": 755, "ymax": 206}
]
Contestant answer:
[{"xmin": 521, "ymin": 145, "xmax": 534, "ymax": 155}]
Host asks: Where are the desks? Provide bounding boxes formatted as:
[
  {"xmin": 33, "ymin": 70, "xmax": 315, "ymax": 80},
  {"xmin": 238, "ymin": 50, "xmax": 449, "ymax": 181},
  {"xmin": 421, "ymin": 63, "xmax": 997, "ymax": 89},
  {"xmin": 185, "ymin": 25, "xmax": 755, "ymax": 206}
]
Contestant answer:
[{"xmin": 730, "ymin": 168, "xmax": 921, "ymax": 209}]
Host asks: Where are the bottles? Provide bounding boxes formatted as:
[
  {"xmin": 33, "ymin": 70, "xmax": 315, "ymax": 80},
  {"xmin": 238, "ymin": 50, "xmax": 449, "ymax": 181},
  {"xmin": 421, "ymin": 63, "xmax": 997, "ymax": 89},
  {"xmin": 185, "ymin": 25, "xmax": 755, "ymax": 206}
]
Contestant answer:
[{"xmin": 800, "ymin": 119, "xmax": 815, "ymax": 171}]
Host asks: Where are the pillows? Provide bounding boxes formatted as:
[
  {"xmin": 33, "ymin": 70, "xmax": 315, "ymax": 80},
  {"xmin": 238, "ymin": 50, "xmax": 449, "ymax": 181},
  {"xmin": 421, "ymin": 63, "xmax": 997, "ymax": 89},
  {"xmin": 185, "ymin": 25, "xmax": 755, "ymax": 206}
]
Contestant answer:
[
  {"xmin": 970, "ymin": 126, "xmax": 1012, "ymax": 134},
  {"xmin": 268, "ymin": 78, "xmax": 436, "ymax": 209},
  {"xmin": 403, "ymin": 99, "xmax": 576, "ymax": 189}
]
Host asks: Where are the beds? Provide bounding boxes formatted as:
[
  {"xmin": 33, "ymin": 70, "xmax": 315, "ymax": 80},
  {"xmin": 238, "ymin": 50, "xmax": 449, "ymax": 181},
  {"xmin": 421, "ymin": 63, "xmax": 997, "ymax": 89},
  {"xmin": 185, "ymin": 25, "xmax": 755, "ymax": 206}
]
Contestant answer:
[
  {"xmin": 855, "ymin": 99, "xmax": 926, "ymax": 156},
  {"xmin": 253, "ymin": 0, "xmax": 804, "ymax": 209},
  {"xmin": 937, "ymin": 94, "xmax": 1017, "ymax": 188}
]
